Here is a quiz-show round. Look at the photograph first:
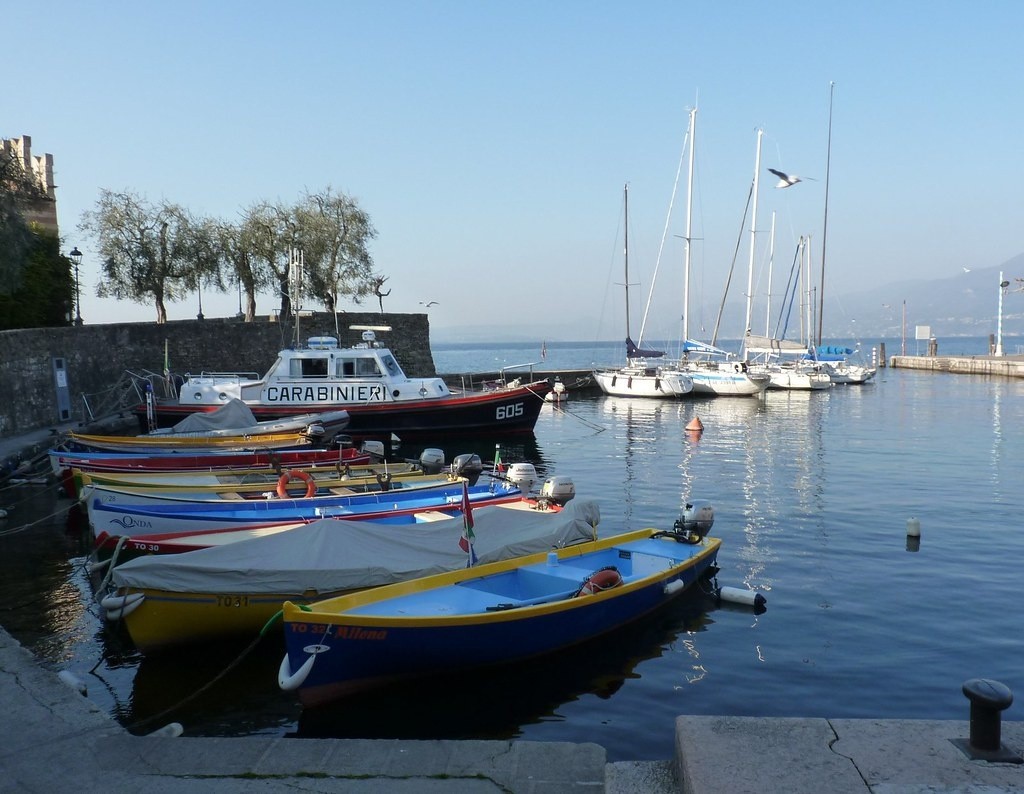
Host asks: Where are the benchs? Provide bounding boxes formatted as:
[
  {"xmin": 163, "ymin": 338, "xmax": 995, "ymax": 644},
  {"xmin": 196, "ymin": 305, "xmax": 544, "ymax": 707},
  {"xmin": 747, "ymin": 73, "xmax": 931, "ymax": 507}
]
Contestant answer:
[
  {"xmin": 217, "ymin": 492, "xmax": 244, "ymax": 501},
  {"xmin": 329, "ymin": 487, "xmax": 357, "ymax": 495},
  {"xmin": 414, "ymin": 511, "xmax": 455, "ymax": 522},
  {"xmin": 310, "ymin": 472, "xmax": 329, "ymax": 480},
  {"xmin": 518, "ymin": 562, "xmax": 627, "ymax": 595},
  {"xmin": 315, "ymin": 506, "xmax": 352, "ymax": 516},
  {"xmin": 215, "ymin": 475, "xmax": 241, "ymax": 484}
]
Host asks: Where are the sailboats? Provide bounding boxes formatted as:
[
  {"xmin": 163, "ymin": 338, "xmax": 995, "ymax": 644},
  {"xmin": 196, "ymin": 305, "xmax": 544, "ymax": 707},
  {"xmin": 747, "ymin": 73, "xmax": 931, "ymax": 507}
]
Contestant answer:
[{"xmin": 589, "ymin": 74, "xmax": 880, "ymax": 398}]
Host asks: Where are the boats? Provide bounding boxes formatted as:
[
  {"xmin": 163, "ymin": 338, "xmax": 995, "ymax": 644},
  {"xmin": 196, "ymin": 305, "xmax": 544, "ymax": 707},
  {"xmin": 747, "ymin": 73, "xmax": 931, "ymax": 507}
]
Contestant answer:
[{"xmin": 42, "ymin": 320, "xmax": 727, "ymax": 716}]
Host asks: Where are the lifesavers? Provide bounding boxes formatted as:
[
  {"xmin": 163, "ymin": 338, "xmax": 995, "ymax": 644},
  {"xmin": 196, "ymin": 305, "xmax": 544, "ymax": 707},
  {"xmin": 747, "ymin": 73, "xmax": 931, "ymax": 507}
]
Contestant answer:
[
  {"xmin": 277, "ymin": 470, "xmax": 316, "ymax": 499},
  {"xmin": 576, "ymin": 569, "xmax": 623, "ymax": 597}
]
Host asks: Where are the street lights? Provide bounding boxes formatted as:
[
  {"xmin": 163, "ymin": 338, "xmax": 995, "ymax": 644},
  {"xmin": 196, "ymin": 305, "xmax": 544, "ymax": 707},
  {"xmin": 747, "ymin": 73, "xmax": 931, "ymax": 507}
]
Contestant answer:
[
  {"xmin": 66, "ymin": 245, "xmax": 85, "ymax": 327},
  {"xmin": 992, "ymin": 268, "xmax": 1012, "ymax": 359}
]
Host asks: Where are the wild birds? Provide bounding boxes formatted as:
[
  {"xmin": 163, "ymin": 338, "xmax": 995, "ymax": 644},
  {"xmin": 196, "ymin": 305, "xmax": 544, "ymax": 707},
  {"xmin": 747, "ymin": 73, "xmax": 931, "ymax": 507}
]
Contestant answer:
[{"xmin": 767, "ymin": 168, "xmax": 816, "ymax": 189}]
